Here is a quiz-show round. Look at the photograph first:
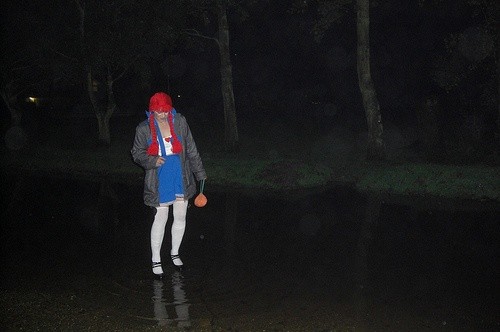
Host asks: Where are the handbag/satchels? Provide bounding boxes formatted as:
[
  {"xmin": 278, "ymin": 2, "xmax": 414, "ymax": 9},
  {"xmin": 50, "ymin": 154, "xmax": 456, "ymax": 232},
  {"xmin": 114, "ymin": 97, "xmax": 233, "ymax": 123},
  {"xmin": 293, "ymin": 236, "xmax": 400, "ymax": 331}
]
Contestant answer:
[{"xmin": 194, "ymin": 179, "xmax": 207, "ymax": 207}]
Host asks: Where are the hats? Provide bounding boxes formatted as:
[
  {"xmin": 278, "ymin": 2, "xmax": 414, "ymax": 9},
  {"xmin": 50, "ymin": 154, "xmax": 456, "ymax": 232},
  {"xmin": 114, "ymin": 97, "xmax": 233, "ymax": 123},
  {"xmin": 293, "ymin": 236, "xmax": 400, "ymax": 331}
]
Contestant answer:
[{"xmin": 148, "ymin": 92, "xmax": 172, "ymax": 113}]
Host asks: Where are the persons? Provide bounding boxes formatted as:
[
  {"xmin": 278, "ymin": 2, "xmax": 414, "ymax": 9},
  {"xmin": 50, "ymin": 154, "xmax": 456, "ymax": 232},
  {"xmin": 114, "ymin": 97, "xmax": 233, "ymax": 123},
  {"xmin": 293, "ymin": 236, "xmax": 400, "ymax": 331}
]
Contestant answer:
[{"xmin": 126, "ymin": 91, "xmax": 209, "ymax": 280}]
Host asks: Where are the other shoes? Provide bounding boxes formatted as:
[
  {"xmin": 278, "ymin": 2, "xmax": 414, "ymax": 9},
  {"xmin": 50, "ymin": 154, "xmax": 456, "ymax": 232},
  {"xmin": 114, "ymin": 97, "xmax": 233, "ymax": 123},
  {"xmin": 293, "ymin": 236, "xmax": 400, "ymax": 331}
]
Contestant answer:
[
  {"xmin": 151, "ymin": 261, "xmax": 165, "ymax": 279},
  {"xmin": 169, "ymin": 255, "xmax": 183, "ymax": 270}
]
